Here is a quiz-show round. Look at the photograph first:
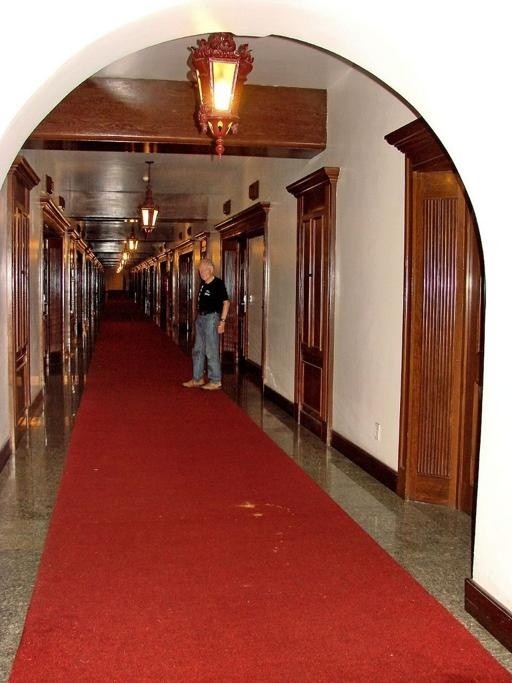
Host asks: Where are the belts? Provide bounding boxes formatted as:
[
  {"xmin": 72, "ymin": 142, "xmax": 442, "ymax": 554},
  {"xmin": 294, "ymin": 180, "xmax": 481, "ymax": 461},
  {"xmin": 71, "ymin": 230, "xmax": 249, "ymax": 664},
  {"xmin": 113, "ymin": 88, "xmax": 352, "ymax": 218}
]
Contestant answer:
[{"xmin": 200, "ymin": 311, "xmax": 213, "ymax": 316}]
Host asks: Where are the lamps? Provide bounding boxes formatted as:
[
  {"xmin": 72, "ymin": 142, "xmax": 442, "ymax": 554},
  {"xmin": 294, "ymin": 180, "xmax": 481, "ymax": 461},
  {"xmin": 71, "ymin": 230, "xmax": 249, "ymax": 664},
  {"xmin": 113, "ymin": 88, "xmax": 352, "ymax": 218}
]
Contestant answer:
[
  {"xmin": 115, "ymin": 223, "xmax": 139, "ymax": 274},
  {"xmin": 187, "ymin": 33, "xmax": 254, "ymax": 161},
  {"xmin": 136, "ymin": 158, "xmax": 161, "ymax": 242}
]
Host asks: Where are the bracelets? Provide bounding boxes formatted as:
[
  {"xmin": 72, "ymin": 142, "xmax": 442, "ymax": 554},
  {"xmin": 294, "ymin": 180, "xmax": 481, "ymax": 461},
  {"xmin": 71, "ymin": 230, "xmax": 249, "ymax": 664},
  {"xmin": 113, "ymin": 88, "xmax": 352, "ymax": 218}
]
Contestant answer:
[{"xmin": 219, "ymin": 317, "xmax": 227, "ymax": 321}]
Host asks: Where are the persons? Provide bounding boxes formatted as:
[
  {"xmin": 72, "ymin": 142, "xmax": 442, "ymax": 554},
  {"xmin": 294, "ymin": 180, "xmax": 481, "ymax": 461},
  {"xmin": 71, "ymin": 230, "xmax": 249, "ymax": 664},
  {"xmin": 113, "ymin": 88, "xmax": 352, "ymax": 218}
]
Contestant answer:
[{"xmin": 182, "ymin": 259, "xmax": 231, "ymax": 389}]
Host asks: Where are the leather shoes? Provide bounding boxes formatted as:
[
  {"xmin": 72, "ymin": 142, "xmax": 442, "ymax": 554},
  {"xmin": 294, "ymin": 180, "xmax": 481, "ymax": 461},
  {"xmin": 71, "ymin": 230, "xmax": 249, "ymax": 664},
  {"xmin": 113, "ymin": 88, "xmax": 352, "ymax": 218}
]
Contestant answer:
[
  {"xmin": 201, "ymin": 382, "xmax": 222, "ymax": 390},
  {"xmin": 182, "ymin": 378, "xmax": 204, "ymax": 387}
]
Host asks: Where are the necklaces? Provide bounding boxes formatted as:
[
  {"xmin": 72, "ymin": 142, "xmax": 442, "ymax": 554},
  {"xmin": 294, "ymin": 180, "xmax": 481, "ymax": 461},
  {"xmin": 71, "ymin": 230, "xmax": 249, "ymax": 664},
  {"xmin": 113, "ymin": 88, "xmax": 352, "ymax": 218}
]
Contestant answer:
[{"xmin": 205, "ymin": 276, "xmax": 214, "ymax": 283}]
